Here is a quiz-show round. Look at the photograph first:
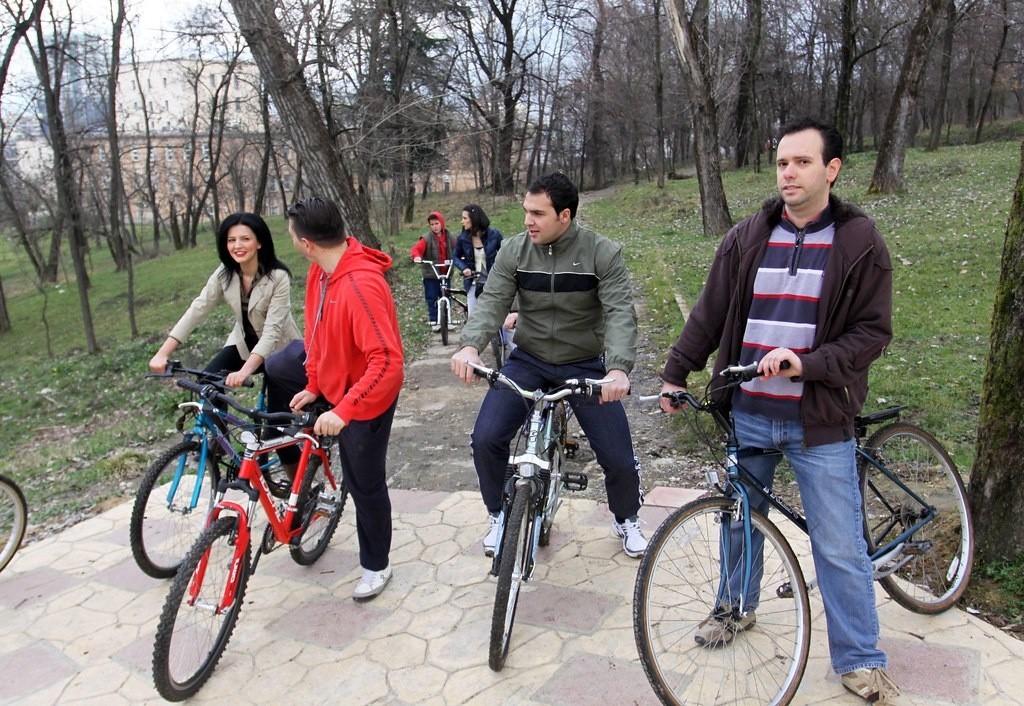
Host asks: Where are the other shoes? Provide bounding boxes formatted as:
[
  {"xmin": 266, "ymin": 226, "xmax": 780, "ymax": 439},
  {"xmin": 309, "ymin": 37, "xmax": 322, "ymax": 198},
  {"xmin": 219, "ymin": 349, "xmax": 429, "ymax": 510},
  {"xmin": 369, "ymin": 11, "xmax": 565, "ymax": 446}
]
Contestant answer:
[{"xmin": 193, "ymin": 430, "xmax": 231, "ymax": 462}]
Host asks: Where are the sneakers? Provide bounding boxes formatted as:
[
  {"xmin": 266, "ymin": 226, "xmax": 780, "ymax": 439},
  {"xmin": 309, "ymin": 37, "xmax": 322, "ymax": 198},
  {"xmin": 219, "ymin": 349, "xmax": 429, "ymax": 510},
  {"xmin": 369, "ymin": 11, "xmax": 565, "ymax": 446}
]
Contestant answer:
[
  {"xmin": 352, "ymin": 558, "xmax": 393, "ymax": 601},
  {"xmin": 695, "ymin": 604, "xmax": 756, "ymax": 644},
  {"xmin": 841, "ymin": 666, "xmax": 912, "ymax": 706},
  {"xmin": 612, "ymin": 515, "xmax": 649, "ymax": 558},
  {"xmin": 482, "ymin": 510, "xmax": 505, "ymax": 557}
]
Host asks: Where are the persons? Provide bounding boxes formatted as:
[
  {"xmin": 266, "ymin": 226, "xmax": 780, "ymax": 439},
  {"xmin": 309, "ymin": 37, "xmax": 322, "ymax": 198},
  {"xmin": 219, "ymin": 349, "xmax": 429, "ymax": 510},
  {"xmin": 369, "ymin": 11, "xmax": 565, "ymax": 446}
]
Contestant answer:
[
  {"xmin": 262, "ymin": 197, "xmax": 405, "ymax": 602},
  {"xmin": 147, "ymin": 212, "xmax": 304, "ymax": 482},
  {"xmin": 659, "ymin": 115, "xmax": 900, "ymax": 702},
  {"xmin": 450, "ymin": 171, "xmax": 648, "ymax": 559},
  {"xmin": 504, "ymin": 290, "xmax": 519, "ymax": 329},
  {"xmin": 411, "ymin": 211, "xmax": 457, "ymax": 333},
  {"xmin": 452, "ymin": 204, "xmax": 518, "ymax": 355}
]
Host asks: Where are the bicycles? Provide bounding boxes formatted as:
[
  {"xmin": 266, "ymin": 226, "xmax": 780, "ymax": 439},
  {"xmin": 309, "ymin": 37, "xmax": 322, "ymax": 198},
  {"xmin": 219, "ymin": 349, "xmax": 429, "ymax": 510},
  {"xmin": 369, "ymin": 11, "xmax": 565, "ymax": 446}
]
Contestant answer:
[
  {"xmin": 132, "ymin": 358, "xmax": 302, "ymax": 580},
  {"xmin": 150, "ymin": 377, "xmax": 348, "ymax": 703},
  {"xmin": 458, "ymin": 271, "xmax": 508, "ymax": 369},
  {"xmin": 630, "ymin": 361, "xmax": 974, "ymax": 706},
  {"xmin": 461, "ymin": 358, "xmax": 617, "ymax": 672},
  {"xmin": 419, "ymin": 257, "xmax": 469, "ymax": 345},
  {"xmin": 0, "ymin": 473, "xmax": 27, "ymax": 573}
]
